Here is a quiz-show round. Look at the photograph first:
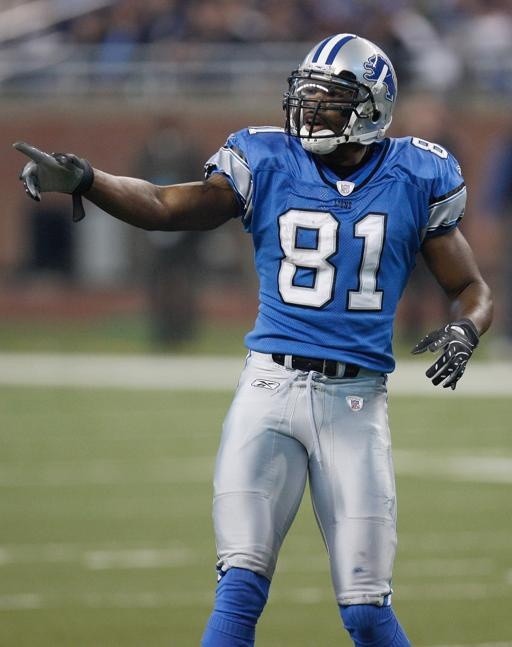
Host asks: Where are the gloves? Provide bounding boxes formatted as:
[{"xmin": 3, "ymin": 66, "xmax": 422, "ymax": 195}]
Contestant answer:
[
  {"xmin": 411, "ymin": 318, "xmax": 481, "ymax": 391},
  {"xmin": 11, "ymin": 142, "xmax": 94, "ymax": 202}
]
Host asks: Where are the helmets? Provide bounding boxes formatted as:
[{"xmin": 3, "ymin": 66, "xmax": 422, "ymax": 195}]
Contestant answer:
[{"xmin": 290, "ymin": 33, "xmax": 398, "ymax": 146}]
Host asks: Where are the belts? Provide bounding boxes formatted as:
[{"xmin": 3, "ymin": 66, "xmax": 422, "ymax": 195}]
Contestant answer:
[{"xmin": 272, "ymin": 353, "xmax": 360, "ymax": 378}]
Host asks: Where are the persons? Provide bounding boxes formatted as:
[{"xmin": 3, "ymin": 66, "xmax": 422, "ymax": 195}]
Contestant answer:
[{"xmin": 12, "ymin": 32, "xmax": 494, "ymax": 647}]
